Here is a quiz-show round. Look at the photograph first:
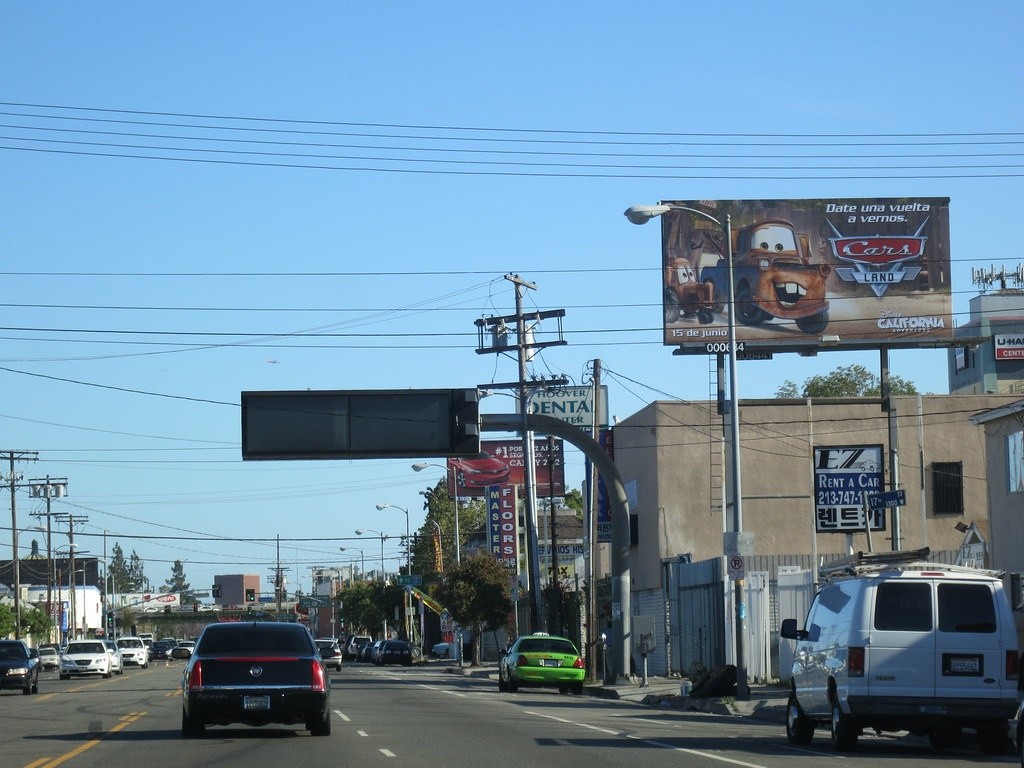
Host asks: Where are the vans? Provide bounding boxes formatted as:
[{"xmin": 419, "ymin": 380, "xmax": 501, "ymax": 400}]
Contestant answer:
[{"xmin": 781, "ymin": 562, "xmax": 1021, "ymax": 752}]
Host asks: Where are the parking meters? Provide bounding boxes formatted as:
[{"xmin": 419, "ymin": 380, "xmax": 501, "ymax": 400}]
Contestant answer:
[{"xmin": 598, "ymin": 633, "xmax": 607, "ymax": 686}]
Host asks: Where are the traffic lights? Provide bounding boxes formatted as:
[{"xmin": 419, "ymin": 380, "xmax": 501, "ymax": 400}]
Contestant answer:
[
  {"xmin": 246, "ymin": 589, "xmax": 255, "ymax": 602},
  {"xmin": 107, "ymin": 612, "xmax": 113, "ymax": 628}
]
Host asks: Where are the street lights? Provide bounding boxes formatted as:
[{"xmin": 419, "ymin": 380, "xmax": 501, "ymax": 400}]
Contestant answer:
[
  {"xmin": 194, "ymin": 602, "xmax": 197, "ymax": 612},
  {"xmin": 341, "ymin": 546, "xmax": 366, "ymax": 636},
  {"xmin": 411, "ymin": 462, "xmax": 463, "ymax": 668},
  {"xmin": 624, "ymin": 202, "xmax": 749, "ymax": 703},
  {"xmin": 355, "ymin": 529, "xmax": 388, "ymax": 640},
  {"xmin": 55, "ymin": 542, "xmax": 79, "ymax": 643},
  {"xmin": 83, "ymin": 559, "xmax": 106, "ymax": 638},
  {"xmin": 375, "ymin": 503, "xmax": 412, "ymax": 643},
  {"xmin": 431, "ymin": 520, "xmax": 442, "ymax": 575},
  {"xmin": 69, "ymin": 570, "xmax": 84, "ymax": 639}
]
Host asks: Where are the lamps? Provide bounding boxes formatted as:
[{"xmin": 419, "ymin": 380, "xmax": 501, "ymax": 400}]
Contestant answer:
[
  {"xmin": 818, "ymin": 333, "xmax": 841, "ymax": 347},
  {"xmin": 954, "ymin": 522, "xmax": 969, "ymax": 533}
]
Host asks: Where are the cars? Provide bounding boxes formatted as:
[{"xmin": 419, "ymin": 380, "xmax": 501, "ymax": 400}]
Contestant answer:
[
  {"xmin": 0, "ymin": 632, "xmax": 198, "ymax": 694},
  {"xmin": 140, "ymin": 606, "xmax": 158, "ymax": 613},
  {"xmin": 181, "ymin": 622, "xmax": 331, "ymax": 736},
  {"xmin": 431, "ymin": 642, "xmax": 451, "ymax": 659},
  {"xmin": 498, "ymin": 632, "xmax": 586, "ymax": 695},
  {"xmin": 447, "ymin": 450, "xmax": 512, "ymax": 487},
  {"xmin": 314, "ymin": 634, "xmax": 422, "ymax": 671},
  {"xmin": 176, "ymin": 604, "xmax": 265, "ymax": 612}
]
